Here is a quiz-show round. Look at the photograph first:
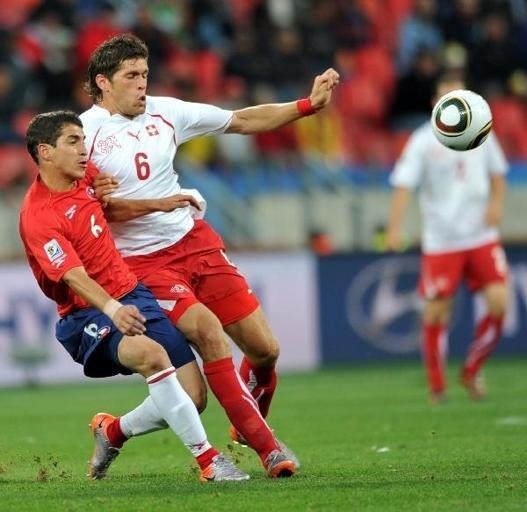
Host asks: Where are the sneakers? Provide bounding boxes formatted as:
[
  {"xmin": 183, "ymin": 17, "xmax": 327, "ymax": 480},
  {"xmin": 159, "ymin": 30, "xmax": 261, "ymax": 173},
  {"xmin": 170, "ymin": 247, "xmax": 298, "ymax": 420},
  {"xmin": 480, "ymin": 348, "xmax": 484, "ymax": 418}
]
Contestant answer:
[
  {"xmin": 86, "ymin": 412, "xmax": 123, "ymax": 481},
  {"xmin": 199, "ymin": 453, "xmax": 250, "ymax": 484},
  {"xmin": 429, "ymin": 376, "xmax": 489, "ymax": 406},
  {"xmin": 263, "ymin": 448, "xmax": 299, "ymax": 478}
]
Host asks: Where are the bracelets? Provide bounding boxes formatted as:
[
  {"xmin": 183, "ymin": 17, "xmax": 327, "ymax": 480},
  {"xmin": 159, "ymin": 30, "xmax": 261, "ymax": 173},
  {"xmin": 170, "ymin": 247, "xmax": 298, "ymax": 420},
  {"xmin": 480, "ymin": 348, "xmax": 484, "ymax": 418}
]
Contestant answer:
[{"xmin": 103, "ymin": 299, "xmax": 123, "ymax": 318}]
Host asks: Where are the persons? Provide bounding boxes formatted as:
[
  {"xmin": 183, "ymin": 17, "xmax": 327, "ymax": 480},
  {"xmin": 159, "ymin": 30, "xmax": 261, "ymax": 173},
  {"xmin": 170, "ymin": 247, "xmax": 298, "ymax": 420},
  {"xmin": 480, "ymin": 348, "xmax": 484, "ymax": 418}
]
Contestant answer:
[
  {"xmin": 72, "ymin": 34, "xmax": 339, "ymax": 475},
  {"xmin": 19, "ymin": 109, "xmax": 250, "ymax": 483},
  {"xmin": 384, "ymin": 73, "xmax": 509, "ymax": 406}
]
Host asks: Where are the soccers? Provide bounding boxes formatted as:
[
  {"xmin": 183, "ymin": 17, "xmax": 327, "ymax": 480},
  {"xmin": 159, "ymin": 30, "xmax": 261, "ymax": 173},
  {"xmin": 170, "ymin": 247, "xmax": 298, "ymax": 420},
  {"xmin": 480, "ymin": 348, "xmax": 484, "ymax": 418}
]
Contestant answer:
[{"xmin": 431, "ymin": 89, "xmax": 492, "ymax": 151}]
